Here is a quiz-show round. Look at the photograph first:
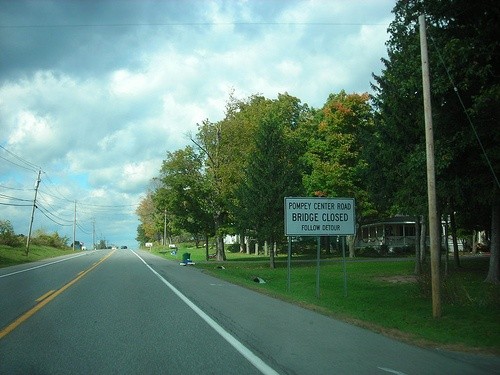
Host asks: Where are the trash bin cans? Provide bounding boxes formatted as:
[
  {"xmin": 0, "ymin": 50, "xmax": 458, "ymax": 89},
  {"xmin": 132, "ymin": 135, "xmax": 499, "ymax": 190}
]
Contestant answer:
[{"xmin": 182, "ymin": 252, "xmax": 190, "ymax": 263}]
